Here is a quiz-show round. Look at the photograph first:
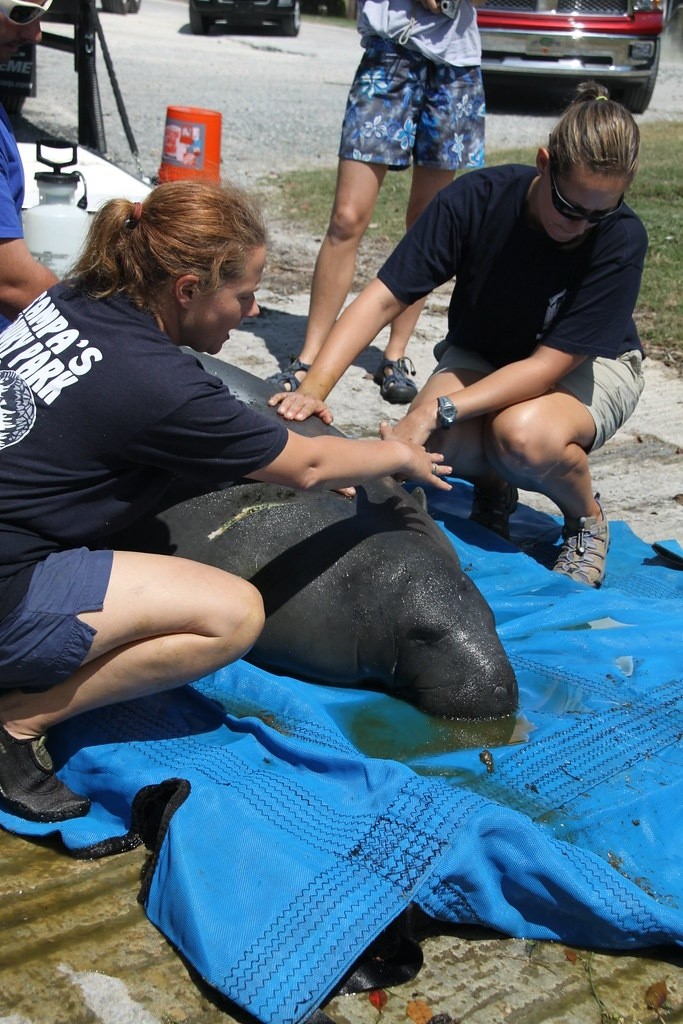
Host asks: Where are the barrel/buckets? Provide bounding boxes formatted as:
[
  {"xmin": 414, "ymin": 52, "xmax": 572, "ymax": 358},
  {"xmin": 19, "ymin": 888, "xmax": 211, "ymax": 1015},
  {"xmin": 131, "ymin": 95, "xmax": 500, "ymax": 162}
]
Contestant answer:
[{"xmin": 158, "ymin": 107, "xmax": 222, "ymax": 190}]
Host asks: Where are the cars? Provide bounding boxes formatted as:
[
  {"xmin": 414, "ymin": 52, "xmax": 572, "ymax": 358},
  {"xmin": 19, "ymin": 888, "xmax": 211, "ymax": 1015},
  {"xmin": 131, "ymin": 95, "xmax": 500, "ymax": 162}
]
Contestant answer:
[{"xmin": 190, "ymin": 0, "xmax": 303, "ymax": 36}]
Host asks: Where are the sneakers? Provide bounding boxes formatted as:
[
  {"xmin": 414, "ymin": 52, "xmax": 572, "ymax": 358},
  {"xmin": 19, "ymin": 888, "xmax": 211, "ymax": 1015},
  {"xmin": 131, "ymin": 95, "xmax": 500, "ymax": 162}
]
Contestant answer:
[
  {"xmin": 465, "ymin": 474, "xmax": 519, "ymax": 542},
  {"xmin": 552, "ymin": 494, "xmax": 609, "ymax": 592},
  {"xmin": 1, "ymin": 723, "xmax": 93, "ymax": 823}
]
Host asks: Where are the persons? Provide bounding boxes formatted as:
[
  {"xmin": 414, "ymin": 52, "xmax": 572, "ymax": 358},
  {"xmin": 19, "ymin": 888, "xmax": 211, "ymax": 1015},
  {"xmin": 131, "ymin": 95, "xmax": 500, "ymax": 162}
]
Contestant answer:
[
  {"xmin": 255, "ymin": 0, "xmax": 488, "ymax": 409},
  {"xmin": 0, "ymin": 1, "xmax": 63, "ymax": 331},
  {"xmin": 266, "ymin": 78, "xmax": 646, "ymax": 588},
  {"xmin": 0, "ymin": 178, "xmax": 455, "ymax": 822}
]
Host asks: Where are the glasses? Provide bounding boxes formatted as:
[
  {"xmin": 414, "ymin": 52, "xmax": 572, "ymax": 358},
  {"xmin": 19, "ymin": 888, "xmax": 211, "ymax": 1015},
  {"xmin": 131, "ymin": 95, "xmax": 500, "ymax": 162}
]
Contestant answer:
[
  {"xmin": 1, "ymin": 0, "xmax": 53, "ymax": 28},
  {"xmin": 549, "ymin": 166, "xmax": 625, "ymax": 224}
]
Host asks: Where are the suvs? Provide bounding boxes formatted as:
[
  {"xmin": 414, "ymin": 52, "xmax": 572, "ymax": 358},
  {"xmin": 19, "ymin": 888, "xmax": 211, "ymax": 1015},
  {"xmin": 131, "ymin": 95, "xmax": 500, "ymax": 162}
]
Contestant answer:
[{"xmin": 473, "ymin": 0, "xmax": 671, "ymax": 114}]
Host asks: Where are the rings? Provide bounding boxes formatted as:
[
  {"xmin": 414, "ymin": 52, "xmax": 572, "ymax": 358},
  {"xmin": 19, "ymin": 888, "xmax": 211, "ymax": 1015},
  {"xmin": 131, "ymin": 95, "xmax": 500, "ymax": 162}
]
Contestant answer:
[{"xmin": 432, "ymin": 462, "xmax": 439, "ymax": 476}]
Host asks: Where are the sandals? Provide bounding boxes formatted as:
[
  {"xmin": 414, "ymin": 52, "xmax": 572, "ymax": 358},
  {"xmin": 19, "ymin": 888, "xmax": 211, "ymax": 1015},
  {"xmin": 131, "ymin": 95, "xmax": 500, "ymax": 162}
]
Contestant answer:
[
  {"xmin": 374, "ymin": 354, "xmax": 419, "ymax": 407},
  {"xmin": 264, "ymin": 357, "xmax": 315, "ymax": 397}
]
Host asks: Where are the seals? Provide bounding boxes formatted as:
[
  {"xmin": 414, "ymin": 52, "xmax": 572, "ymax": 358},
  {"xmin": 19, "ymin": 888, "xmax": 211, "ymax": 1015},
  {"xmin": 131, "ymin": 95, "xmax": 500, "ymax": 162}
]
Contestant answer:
[{"xmin": 97, "ymin": 342, "xmax": 521, "ymax": 724}]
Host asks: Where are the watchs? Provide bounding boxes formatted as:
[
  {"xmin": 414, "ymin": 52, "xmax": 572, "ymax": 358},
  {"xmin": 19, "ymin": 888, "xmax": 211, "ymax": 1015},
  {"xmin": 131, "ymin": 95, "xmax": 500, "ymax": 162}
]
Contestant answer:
[{"xmin": 436, "ymin": 395, "xmax": 459, "ymax": 431}]
